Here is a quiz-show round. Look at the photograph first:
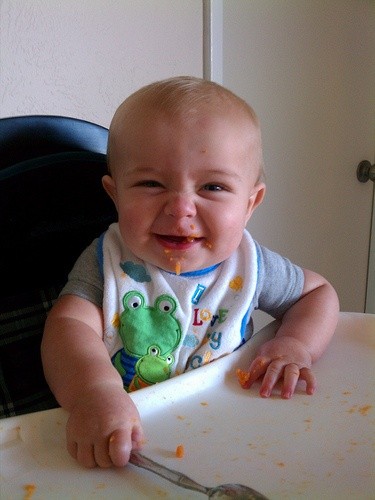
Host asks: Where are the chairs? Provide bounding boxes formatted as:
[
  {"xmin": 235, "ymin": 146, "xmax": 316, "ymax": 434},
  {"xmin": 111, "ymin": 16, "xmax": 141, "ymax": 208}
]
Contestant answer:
[{"xmin": 0, "ymin": 115, "xmax": 119, "ymax": 419}]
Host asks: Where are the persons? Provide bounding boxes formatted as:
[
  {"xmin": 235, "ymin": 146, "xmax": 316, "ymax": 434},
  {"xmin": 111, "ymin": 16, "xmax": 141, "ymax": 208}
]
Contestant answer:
[{"xmin": 41, "ymin": 77, "xmax": 339, "ymax": 469}]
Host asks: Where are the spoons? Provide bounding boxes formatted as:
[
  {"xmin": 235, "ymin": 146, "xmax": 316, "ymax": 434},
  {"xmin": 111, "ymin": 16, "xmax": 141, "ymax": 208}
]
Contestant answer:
[{"xmin": 128, "ymin": 452, "xmax": 269, "ymax": 500}]
